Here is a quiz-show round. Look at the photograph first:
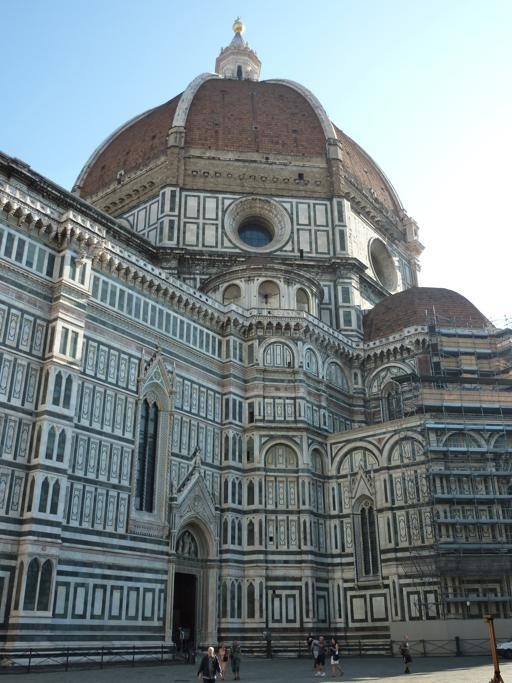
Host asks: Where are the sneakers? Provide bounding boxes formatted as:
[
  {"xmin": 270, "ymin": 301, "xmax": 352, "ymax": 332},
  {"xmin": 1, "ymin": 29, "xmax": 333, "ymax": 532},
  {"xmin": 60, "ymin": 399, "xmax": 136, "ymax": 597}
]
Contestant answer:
[{"xmin": 314, "ymin": 671, "xmax": 344, "ymax": 678}]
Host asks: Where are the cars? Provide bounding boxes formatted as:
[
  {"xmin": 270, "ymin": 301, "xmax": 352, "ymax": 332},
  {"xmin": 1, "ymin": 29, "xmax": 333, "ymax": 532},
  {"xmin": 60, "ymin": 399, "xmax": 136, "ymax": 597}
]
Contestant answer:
[{"xmin": 496, "ymin": 641, "xmax": 512, "ymax": 660}]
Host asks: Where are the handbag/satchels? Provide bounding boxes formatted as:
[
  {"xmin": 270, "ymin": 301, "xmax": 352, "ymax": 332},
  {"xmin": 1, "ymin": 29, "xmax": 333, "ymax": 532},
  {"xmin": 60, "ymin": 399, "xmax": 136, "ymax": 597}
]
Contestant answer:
[
  {"xmin": 333, "ymin": 654, "xmax": 341, "ymax": 661},
  {"xmin": 224, "ymin": 656, "xmax": 228, "ymax": 661}
]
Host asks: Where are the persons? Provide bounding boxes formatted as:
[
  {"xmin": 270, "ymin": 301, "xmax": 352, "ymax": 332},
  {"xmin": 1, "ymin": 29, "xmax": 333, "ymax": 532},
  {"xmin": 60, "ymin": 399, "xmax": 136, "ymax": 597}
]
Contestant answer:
[
  {"xmin": 307, "ymin": 636, "xmax": 345, "ymax": 678},
  {"xmin": 399, "ymin": 633, "xmax": 413, "ymax": 674},
  {"xmin": 176, "ymin": 626, "xmax": 185, "ymax": 653},
  {"xmin": 197, "ymin": 639, "xmax": 240, "ymax": 683}
]
